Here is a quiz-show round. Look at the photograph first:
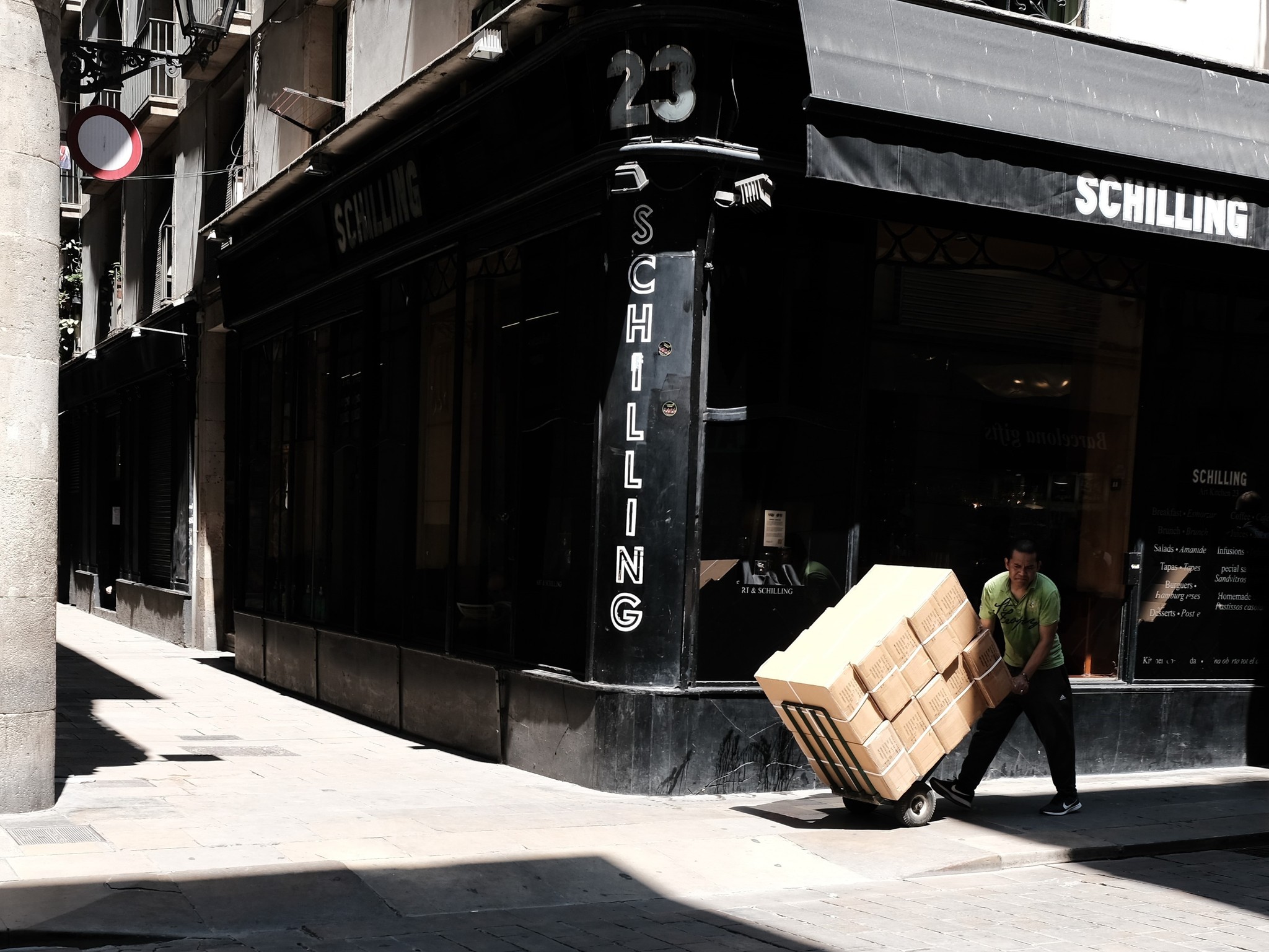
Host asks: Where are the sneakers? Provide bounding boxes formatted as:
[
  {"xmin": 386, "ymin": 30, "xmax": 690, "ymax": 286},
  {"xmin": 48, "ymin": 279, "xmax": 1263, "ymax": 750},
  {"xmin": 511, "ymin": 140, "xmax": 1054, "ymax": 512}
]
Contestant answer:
[
  {"xmin": 930, "ymin": 776, "xmax": 975, "ymax": 808},
  {"xmin": 1041, "ymin": 788, "xmax": 1081, "ymax": 815}
]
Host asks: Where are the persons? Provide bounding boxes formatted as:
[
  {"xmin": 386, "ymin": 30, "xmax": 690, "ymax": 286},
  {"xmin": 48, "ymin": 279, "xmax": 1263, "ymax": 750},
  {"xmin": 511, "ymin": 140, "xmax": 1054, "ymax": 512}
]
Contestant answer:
[{"xmin": 929, "ymin": 538, "xmax": 1083, "ymax": 817}]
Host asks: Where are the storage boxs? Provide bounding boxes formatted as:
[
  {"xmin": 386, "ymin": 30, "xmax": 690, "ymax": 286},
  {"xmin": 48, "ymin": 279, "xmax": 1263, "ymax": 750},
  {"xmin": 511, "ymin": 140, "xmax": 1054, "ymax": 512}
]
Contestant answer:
[{"xmin": 754, "ymin": 564, "xmax": 1014, "ymax": 801}]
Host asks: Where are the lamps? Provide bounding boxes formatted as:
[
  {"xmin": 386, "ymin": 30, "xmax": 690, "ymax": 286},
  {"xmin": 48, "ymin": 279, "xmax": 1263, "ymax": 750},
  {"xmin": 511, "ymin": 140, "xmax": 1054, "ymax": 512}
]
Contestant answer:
[
  {"xmin": 468, "ymin": 28, "xmax": 504, "ymax": 62},
  {"xmin": 206, "ymin": 224, "xmax": 230, "ymax": 243},
  {"xmin": 734, "ymin": 174, "xmax": 776, "ymax": 214},
  {"xmin": 304, "ymin": 152, "xmax": 332, "ymax": 176},
  {"xmin": 611, "ymin": 161, "xmax": 649, "ymax": 194}
]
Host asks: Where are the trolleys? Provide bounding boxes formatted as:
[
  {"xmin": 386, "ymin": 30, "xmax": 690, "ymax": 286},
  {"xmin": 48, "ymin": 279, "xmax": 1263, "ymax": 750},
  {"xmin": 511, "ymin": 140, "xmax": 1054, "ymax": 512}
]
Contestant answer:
[{"xmin": 780, "ymin": 701, "xmax": 948, "ymax": 827}]
